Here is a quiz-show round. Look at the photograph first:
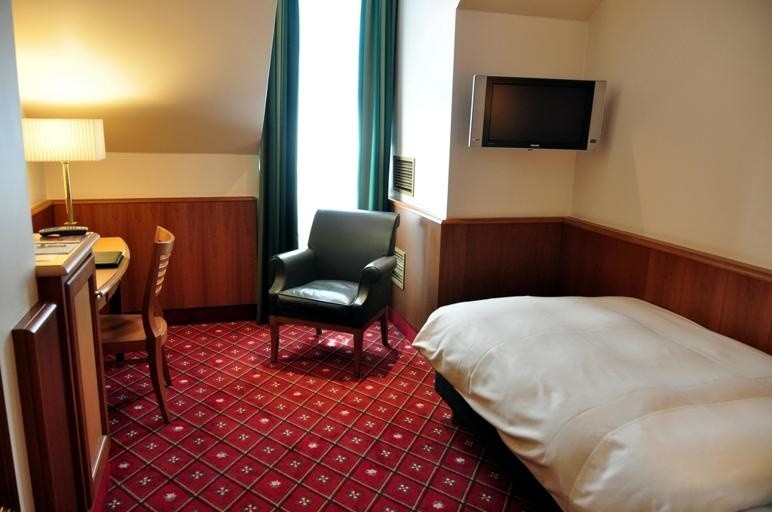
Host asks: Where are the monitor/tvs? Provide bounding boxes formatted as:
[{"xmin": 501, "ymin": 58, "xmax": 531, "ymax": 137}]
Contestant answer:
[{"xmin": 468, "ymin": 74, "xmax": 608, "ymax": 152}]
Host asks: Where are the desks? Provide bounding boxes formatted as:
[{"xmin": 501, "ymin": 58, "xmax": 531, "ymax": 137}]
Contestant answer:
[{"xmin": 93, "ymin": 235, "xmax": 132, "ymax": 367}]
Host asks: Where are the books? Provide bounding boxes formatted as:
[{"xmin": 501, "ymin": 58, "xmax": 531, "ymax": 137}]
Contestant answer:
[{"xmin": 35, "ymin": 243, "xmax": 79, "ymax": 255}]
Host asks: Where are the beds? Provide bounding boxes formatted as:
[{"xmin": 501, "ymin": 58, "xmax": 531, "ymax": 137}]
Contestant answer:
[{"xmin": 410, "ymin": 295, "xmax": 772, "ymax": 511}]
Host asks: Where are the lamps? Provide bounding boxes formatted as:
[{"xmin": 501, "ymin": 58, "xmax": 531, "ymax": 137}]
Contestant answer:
[{"xmin": 22, "ymin": 116, "xmax": 107, "ymax": 227}]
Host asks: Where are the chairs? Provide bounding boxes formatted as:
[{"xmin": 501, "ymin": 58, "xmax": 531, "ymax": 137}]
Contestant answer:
[
  {"xmin": 267, "ymin": 208, "xmax": 400, "ymax": 379},
  {"xmin": 97, "ymin": 226, "xmax": 177, "ymax": 425}
]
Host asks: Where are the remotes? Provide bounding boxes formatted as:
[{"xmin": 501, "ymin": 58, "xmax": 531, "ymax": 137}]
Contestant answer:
[{"xmin": 38, "ymin": 225, "xmax": 89, "ymax": 237}]
